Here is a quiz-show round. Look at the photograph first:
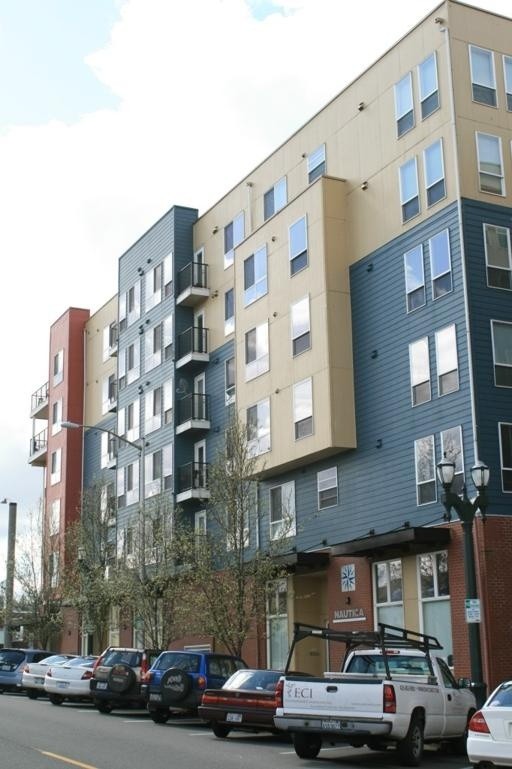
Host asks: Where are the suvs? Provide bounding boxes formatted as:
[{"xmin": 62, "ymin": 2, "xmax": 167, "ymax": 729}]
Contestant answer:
[
  {"xmin": 90, "ymin": 646, "xmax": 167, "ymax": 715},
  {"xmin": 140, "ymin": 648, "xmax": 251, "ymax": 722}
]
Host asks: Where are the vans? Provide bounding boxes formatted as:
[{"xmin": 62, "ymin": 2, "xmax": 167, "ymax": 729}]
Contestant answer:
[{"xmin": 0, "ymin": 648, "xmax": 64, "ymax": 695}]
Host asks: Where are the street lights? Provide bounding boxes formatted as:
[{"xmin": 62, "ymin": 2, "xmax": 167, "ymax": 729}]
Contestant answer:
[
  {"xmin": 59, "ymin": 422, "xmax": 148, "ymax": 650},
  {"xmin": 435, "ymin": 450, "xmax": 492, "ymax": 711}
]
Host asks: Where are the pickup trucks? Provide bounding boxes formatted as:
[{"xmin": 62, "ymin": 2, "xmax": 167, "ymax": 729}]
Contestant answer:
[{"xmin": 272, "ymin": 621, "xmax": 478, "ymax": 767}]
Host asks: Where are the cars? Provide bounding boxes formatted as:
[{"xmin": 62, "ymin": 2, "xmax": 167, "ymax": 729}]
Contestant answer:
[
  {"xmin": 465, "ymin": 679, "xmax": 512, "ymax": 768},
  {"xmin": 196, "ymin": 669, "xmax": 319, "ymax": 742},
  {"xmin": 20, "ymin": 654, "xmax": 79, "ymax": 700},
  {"xmin": 43, "ymin": 654, "xmax": 102, "ymax": 706}
]
